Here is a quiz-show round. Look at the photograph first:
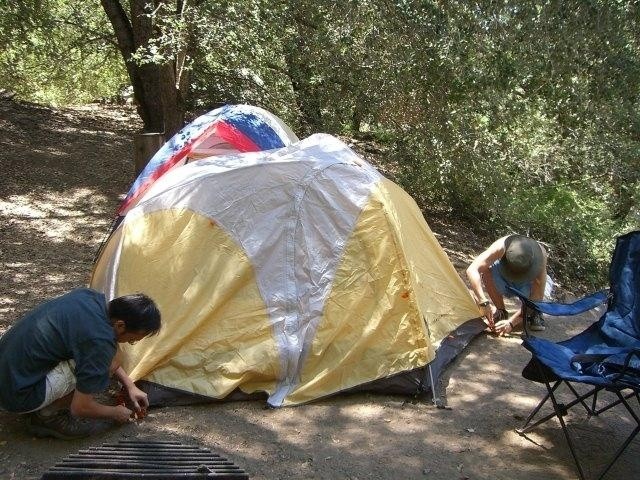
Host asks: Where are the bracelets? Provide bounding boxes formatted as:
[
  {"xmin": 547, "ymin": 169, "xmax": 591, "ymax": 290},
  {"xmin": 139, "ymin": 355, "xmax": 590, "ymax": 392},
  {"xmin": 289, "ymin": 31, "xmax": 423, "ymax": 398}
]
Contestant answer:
[
  {"xmin": 479, "ymin": 301, "xmax": 489, "ymax": 307},
  {"xmin": 466, "ymin": 236, "xmax": 548, "ymax": 336}
]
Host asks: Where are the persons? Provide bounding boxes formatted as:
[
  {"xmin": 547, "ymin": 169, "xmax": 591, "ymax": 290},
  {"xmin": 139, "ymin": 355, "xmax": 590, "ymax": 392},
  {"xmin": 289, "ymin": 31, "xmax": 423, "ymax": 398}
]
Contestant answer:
[{"xmin": 0, "ymin": 286, "xmax": 162, "ymax": 441}]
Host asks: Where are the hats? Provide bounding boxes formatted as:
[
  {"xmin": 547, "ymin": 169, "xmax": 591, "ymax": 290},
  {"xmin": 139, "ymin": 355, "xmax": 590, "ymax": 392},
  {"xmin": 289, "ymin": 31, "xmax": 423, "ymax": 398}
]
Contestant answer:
[{"xmin": 499, "ymin": 235, "xmax": 544, "ymax": 283}]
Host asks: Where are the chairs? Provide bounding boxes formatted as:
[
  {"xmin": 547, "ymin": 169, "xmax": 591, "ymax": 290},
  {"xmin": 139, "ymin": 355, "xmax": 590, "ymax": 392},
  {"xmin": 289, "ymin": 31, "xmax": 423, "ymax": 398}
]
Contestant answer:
[{"xmin": 504, "ymin": 231, "xmax": 640, "ymax": 479}]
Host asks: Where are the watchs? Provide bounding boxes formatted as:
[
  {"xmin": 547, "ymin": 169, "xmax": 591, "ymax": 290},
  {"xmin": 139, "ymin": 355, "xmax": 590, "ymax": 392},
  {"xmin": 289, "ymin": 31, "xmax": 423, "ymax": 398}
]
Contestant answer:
[{"xmin": 509, "ymin": 321, "xmax": 515, "ymax": 329}]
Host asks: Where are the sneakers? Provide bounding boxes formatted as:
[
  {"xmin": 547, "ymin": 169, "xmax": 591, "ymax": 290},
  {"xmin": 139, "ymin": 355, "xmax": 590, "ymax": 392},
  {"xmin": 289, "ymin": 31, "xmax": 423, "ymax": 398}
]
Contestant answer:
[
  {"xmin": 24, "ymin": 408, "xmax": 97, "ymax": 440},
  {"xmin": 493, "ymin": 309, "xmax": 508, "ymax": 323},
  {"xmin": 527, "ymin": 311, "xmax": 545, "ymax": 330}
]
